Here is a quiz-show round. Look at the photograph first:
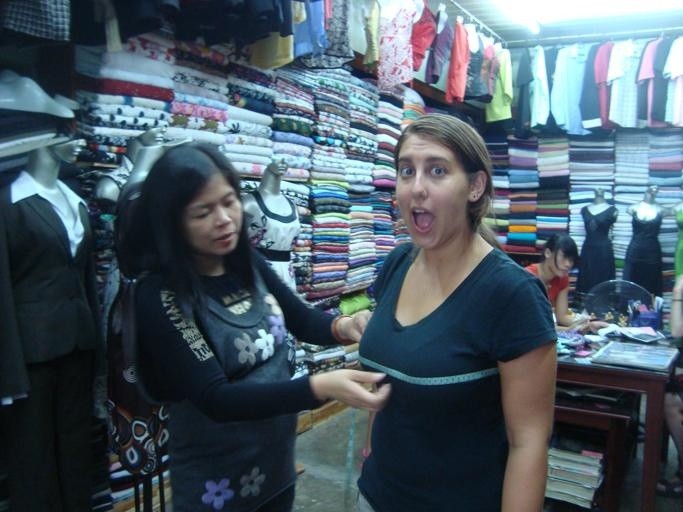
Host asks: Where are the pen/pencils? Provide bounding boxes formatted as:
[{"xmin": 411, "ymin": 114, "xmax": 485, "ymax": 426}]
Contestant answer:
[
  {"xmin": 657, "ymin": 301, "xmax": 664, "ymax": 315},
  {"xmin": 628, "ymin": 305, "xmax": 639, "ymax": 321}
]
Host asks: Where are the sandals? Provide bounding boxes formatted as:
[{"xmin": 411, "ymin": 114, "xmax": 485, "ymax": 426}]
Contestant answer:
[{"xmin": 656, "ymin": 472, "xmax": 681, "ymax": 500}]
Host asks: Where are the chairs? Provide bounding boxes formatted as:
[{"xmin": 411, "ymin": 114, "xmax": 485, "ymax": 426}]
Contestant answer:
[{"xmin": 580, "ymin": 279, "xmax": 651, "ymax": 314}]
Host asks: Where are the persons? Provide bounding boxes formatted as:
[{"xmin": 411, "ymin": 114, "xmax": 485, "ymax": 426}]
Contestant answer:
[
  {"xmin": 114, "ymin": 141, "xmax": 162, "ymax": 414},
  {"xmin": 622, "ymin": 184, "xmax": 665, "ymax": 309},
  {"xmin": 526, "ymin": 233, "xmax": 612, "ymax": 333},
  {"xmin": 129, "ymin": 140, "xmax": 393, "ymax": 512},
  {"xmin": 356, "ymin": 112, "xmax": 557, "ymax": 512},
  {"xmin": 242, "ymin": 157, "xmax": 304, "ymax": 377},
  {"xmin": 573, "ymin": 188, "xmax": 619, "ymax": 314},
  {"xmin": 673, "ymin": 182, "xmax": 682, "ymax": 286},
  {"xmin": 656, "ymin": 274, "xmax": 683, "ymax": 500},
  {"xmin": 87, "ymin": 121, "xmax": 169, "ymax": 477},
  {"xmin": 1, "ymin": 139, "xmax": 110, "ymax": 511}
]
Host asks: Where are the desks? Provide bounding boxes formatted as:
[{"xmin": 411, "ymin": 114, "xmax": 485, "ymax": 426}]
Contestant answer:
[{"xmin": 554, "ymin": 343, "xmax": 679, "ymax": 512}]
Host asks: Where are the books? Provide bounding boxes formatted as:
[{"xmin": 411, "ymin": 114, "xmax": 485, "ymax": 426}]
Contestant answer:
[
  {"xmin": 544, "ymin": 431, "xmax": 608, "ymax": 509},
  {"xmin": 617, "ymin": 326, "xmax": 663, "ymax": 343},
  {"xmin": 592, "ymin": 340, "xmax": 680, "ymax": 371}
]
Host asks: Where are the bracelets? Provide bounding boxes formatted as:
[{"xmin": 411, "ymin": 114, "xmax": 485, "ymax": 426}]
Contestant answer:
[
  {"xmin": 330, "ymin": 314, "xmax": 355, "ymax": 345},
  {"xmin": 672, "ymin": 299, "xmax": 682, "ymax": 303}
]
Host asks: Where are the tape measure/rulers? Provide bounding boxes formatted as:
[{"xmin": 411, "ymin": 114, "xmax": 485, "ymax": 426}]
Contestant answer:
[{"xmin": 343, "ymin": 354, "xmax": 500, "ymax": 512}]
